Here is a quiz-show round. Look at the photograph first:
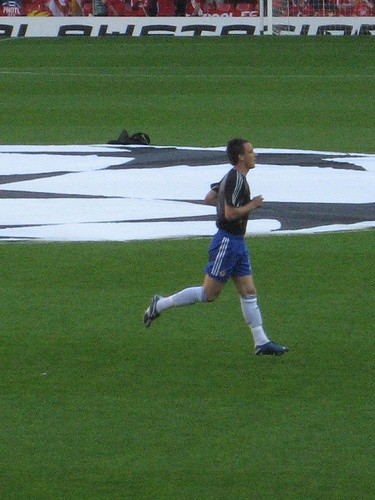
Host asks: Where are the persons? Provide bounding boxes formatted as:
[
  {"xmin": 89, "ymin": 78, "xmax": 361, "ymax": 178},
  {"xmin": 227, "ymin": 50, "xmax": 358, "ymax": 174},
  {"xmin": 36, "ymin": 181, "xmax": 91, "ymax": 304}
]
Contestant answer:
[
  {"xmin": 143, "ymin": 139, "xmax": 289, "ymax": 356},
  {"xmin": 0, "ymin": 0, "xmax": 375, "ymax": 17}
]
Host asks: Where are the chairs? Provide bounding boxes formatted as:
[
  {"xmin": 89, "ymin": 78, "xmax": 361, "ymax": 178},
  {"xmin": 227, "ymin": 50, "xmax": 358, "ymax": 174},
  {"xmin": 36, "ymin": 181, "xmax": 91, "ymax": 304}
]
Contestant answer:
[{"xmin": 185, "ymin": 3, "xmax": 254, "ymax": 16}]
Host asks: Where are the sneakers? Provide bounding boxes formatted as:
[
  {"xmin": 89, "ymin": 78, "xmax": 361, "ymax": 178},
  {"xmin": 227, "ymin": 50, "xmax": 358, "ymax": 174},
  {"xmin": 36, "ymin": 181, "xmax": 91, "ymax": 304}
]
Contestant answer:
[
  {"xmin": 256, "ymin": 341, "xmax": 289, "ymax": 356},
  {"xmin": 144, "ymin": 295, "xmax": 159, "ymax": 328}
]
